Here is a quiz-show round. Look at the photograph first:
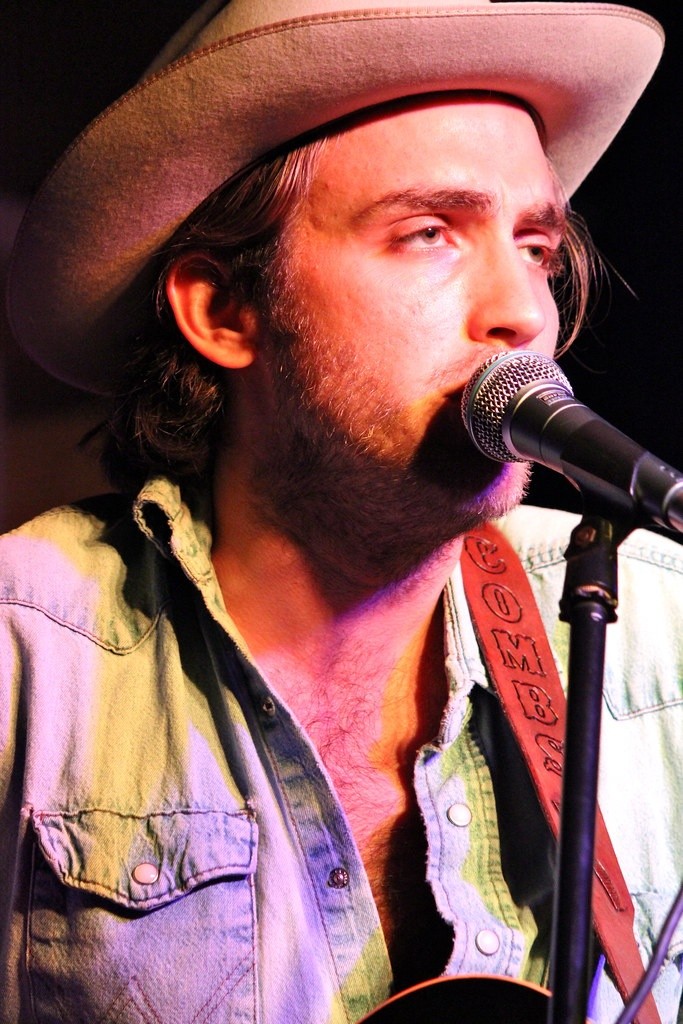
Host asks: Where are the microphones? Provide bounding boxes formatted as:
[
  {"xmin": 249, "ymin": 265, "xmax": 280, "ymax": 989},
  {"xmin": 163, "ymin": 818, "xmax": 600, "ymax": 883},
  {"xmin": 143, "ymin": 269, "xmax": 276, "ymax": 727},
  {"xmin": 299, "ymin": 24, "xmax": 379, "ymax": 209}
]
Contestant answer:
[{"xmin": 460, "ymin": 349, "xmax": 683, "ymax": 536}]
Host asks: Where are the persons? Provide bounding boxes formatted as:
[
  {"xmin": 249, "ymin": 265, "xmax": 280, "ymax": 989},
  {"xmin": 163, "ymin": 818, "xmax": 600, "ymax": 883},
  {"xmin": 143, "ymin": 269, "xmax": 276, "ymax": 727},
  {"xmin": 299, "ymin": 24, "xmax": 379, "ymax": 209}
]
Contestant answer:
[{"xmin": 0, "ymin": 0, "xmax": 683, "ymax": 1024}]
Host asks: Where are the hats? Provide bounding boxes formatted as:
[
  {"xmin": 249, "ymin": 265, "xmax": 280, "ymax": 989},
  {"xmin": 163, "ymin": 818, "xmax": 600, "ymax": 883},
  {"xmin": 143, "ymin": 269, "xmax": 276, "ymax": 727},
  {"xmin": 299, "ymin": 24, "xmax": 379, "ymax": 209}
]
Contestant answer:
[{"xmin": 1, "ymin": 0, "xmax": 666, "ymax": 381}]
